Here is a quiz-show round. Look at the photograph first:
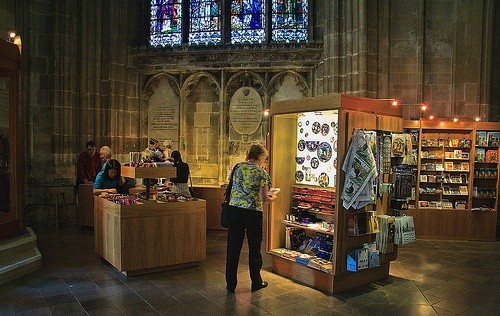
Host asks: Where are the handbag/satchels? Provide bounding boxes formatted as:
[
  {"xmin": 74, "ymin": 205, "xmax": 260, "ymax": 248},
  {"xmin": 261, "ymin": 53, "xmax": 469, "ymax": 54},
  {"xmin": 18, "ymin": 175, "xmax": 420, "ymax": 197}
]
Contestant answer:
[
  {"xmin": 190, "ymin": 187, "xmax": 199, "ymax": 198},
  {"xmin": 221, "ymin": 201, "xmax": 231, "ymax": 228}
]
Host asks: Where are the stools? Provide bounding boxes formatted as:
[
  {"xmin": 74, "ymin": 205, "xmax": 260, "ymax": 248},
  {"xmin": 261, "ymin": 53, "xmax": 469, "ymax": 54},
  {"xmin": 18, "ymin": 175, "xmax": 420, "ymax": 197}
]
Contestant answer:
[{"xmin": 47, "ymin": 192, "xmax": 67, "ymax": 227}]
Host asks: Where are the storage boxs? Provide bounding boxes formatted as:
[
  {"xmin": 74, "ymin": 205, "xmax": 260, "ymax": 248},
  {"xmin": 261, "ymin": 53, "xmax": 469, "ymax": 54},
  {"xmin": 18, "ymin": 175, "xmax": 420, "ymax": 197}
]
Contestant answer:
[{"xmin": 355, "ymin": 248, "xmax": 369, "ymax": 269}]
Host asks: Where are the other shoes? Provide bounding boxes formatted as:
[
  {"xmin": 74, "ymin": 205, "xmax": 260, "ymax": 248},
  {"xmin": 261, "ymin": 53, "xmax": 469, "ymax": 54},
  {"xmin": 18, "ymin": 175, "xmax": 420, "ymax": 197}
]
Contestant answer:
[
  {"xmin": 252, "ymin": 281, "xmax": 268, "ymax": 291},
  {"xmin": 227, "ymin": 289, "xmax": 234, "ymax": 293}
]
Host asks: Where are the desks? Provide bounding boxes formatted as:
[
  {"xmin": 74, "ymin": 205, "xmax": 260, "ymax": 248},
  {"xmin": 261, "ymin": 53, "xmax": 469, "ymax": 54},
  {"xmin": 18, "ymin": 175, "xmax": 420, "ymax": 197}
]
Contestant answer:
[{"xmin": 79, "ymin": 184, "xmax": 94, "ymax": 229}]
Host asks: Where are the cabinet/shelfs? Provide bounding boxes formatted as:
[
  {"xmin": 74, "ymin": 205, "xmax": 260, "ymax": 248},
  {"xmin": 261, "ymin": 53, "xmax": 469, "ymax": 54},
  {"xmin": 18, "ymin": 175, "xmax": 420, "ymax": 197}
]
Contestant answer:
[{"xmin": 94, "ymin": 95, "xmax": 500, "ymax": 294}]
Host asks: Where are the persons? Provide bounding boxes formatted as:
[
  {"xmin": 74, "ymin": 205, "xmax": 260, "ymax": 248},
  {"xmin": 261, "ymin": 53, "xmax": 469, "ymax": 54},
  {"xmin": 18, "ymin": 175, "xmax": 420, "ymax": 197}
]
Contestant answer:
[
  {"xmin": 90, "ymin": 145, "xmax": 112, "ymax": 185},
  {"xmin": 92, "ymin": 159, "xmax": 126, "ymax": 194},
  {"xmin": 226, "ymin": 143, "xmax": 278, "ymax": 293},
  {"xmin": 76, "ymin": 141, "xmax": 107, "ymax": 202},
  {"xmin": 169, "ymin": 150, "xmax": 193, "ymax": 197}
]
